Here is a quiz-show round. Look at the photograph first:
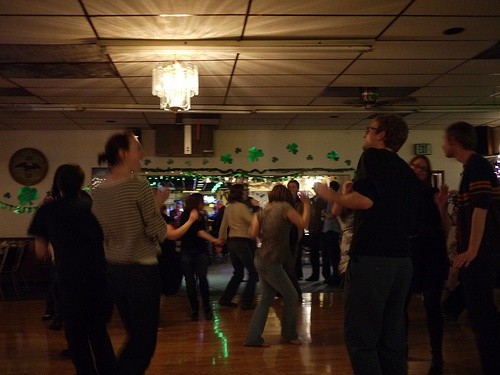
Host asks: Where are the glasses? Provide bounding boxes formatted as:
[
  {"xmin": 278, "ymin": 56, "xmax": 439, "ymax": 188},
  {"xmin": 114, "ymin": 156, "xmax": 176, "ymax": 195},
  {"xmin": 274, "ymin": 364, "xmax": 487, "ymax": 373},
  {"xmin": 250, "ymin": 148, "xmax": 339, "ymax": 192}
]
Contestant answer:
[
  {"xmin": 365, "ymin": 126, "xmax": 378, "ymax": 134},
  {"xmin": 412, "ymin": 163, "xmax": 430, "ymax": 173},
  {"xmin": 243, "ymin": 189, "xmax": 249, "ymax": 191}
]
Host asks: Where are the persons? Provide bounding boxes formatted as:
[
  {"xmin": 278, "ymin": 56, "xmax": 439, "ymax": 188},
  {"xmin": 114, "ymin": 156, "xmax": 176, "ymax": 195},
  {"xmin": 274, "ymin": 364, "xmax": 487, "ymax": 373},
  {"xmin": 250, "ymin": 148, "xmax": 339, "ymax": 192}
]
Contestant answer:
[
  {"xmin": 92, "ymin": 132, "xmax": 169, "ymax": 375},
  {"xmin": 42, "ymin": 298, "xmax": 71, "ymax": 360},
  {"xmin": 28, "ymin": 164, "xmax": 116, "ymax": 375},
  {"xmin": 158, "ymin": 180, "xmax": 354, "ymax": 346},
  {"xmin": 409, "ymin": 120, "xmax": 500, "ymax": 375},
  {"xmin": 313, "ymin": 114, "xmax": 423, "ymax": 375}
]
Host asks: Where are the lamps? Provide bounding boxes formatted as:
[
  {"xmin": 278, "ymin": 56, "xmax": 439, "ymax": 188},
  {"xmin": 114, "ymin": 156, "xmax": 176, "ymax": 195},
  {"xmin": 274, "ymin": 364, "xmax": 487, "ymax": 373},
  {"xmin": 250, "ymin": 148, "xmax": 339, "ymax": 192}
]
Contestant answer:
[
  {"xmin": 98, "ymin": 38, "xmax": 377, "ymax": 54},
  {"xmin": 152, "ymin": 62, "xmax": 199, "ymax": 112},
  {"xmin": 0, "ymin": 104, "xmax": 500, "ymax": 113}
]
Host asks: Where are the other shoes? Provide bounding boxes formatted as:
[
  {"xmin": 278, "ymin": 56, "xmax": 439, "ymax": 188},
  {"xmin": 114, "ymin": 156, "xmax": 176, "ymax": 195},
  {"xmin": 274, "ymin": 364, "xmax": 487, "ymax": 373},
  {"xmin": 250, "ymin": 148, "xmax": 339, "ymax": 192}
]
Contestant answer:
[
  {"xmin": 242, "ymin": 338, "xmax": 270, "ymax": 347},
  {"xmin": 305, "ymin": 273, "xmax": 319, "ymax": 281},
  {"xmin": 242, "ymin": 302, "xmax": 258, "ymax": 310},
  {"xmin": 41, "ymin": 309, "xmax": 53, "ymax": 321},
  {"xmin": 427, "ymin": 360, "xmax": 444, "ymax": 375},
  {"xmin": 281, "ymin": 334, "xmax": 302, "ymax": 345},
  {"xmin": 60, "ymin": 348, "xmax": 70, "ymax": 357},
  {"xmin": 48, "ymin": 319, "xmax": 63, "ymax": 331},
  {"xmin": 192, "ymin": 309, "xmax": 200, "ymax": 321},
  {"xmin": 219, "ymin": 299, "xmax": 238, "ymax": 307},
  {"xmin": 323, "ymin": 276, "xmax": 340, "ymax": 284},
  {"xmin": 204, "ymin": 308, "xmax": 213, "ymax": 320}
]
